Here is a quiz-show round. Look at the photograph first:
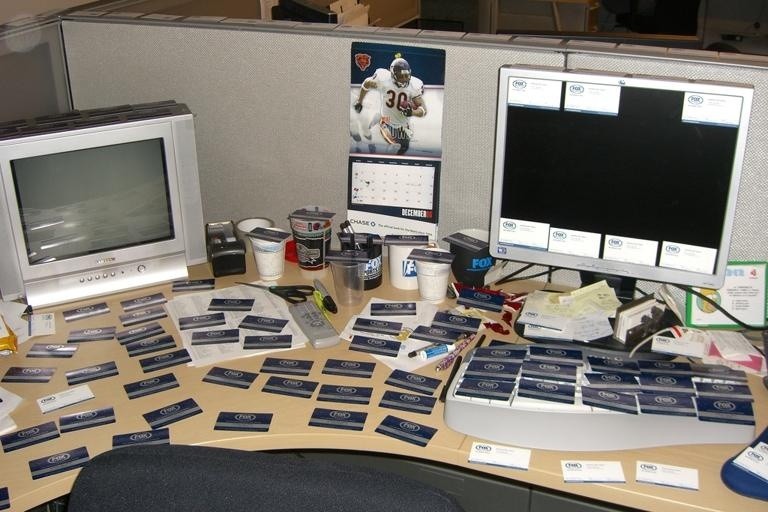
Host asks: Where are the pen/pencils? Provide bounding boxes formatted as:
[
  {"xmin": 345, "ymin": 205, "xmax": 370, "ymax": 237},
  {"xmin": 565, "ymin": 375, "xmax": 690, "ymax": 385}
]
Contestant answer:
[
  {"xmin": 438, "ymin": 356, "xmax": 462, "ymax": 403},
  {"xmin": 434, "ymin": 333, "xmax": 476, "ymax": 372},
  {"xmin": 408, "ymin": 341, "xmax": 441, "ymax": 358},
  {"xmin": 474, "ymin": 335, "xmax": 486, "ymax": 349}
]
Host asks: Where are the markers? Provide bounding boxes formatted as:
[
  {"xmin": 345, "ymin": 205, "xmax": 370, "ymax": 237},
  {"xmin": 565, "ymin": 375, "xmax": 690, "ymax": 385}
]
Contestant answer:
[
  {"xmin": 314, "ymin": 278, "xmax": 338, "ymax": 314},
  {"xmin": 339, "ymin": 220, "xmax": 361, "ymax": 250},
  {"xmin": 366, "ymin": 234, "xmax": 374, "ymax": 259}
]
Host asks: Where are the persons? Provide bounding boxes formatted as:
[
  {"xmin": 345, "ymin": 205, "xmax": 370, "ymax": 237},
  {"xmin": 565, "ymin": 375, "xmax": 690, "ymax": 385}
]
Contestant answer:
[{"xmin": 355, "ymin": 57, "xmax": 427, "ymax": 155}]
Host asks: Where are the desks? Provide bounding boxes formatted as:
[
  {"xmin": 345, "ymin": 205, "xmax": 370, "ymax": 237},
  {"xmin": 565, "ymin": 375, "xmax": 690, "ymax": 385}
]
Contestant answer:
[{"xmin": 0, "ymin": 14, "xmax": 768, "ymax": 512}]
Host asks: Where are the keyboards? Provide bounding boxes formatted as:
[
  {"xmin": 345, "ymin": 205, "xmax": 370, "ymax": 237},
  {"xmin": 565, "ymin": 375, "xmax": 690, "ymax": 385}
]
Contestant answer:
[{"xmin": 443, "ymin": 346, "xmax": 756, "ymax": 452}]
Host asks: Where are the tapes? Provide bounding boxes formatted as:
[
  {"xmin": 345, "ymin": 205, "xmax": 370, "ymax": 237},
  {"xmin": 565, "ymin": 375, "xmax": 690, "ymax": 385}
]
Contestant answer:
[{"xmin": 233, "ymin": 216, "xmax": 275, "ymax": 248}]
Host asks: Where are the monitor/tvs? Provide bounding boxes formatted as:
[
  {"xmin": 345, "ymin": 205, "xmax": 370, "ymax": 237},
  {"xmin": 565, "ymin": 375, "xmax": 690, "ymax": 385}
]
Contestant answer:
[
  {"xmin": 487, "ymin": 63, "xmax": 754, "ymax": 359},
  {"xmin": 0, "ymin": 103, "xmax": 208, "ymax": 311}
]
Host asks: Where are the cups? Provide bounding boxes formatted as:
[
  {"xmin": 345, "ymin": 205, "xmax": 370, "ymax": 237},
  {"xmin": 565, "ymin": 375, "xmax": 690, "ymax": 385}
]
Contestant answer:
[
  {"xmin": 449, "ymin": 228, "xmax": 498, "ymax": 291},
  {"xmin": 388, "ymin": 240, "xmax": 440, "ymax": 291},
  {"xmin": 289, "ymin": 223, "xmax": 333, "ymax": 272},
  {"xmin": 341, "ymin": 233, "xmax": 383, "ymax": 291},
  {"xmin": 248, "ymin": 227, "xmax": 287, "ymax": 281},
  {"xmin": 330, "ymin": 249, "xmax": 366, "ymax": 307},
  {"xmin": 415, "ymin": 247, "xmax": 451, "ymax": 306}
]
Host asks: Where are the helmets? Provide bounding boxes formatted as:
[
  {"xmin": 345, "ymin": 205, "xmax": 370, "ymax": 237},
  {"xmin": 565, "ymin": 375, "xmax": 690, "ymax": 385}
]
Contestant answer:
[{"xmin": 390, "ymin": 57, "xmax": 412, "ymax": 88}]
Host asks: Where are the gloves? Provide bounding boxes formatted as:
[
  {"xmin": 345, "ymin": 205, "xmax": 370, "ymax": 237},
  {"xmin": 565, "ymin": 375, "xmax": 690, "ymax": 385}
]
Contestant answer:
[
  {"xmin": 354, "ymin": 101, "xmax": 363, "ymax": 114},
  {"xmin": 399, "ymin": 102, "xmax": 412, "ymax": 117}
]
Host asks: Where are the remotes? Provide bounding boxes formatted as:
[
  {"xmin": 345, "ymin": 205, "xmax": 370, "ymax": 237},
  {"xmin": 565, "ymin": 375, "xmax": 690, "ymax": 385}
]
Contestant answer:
[{"xmin": 289, "ymin": 300, "xmax": 341, "ymax": 348}]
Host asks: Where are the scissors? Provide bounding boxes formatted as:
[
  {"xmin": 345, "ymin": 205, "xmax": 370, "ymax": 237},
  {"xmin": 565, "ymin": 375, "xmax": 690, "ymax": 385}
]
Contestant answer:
[{"xmin": 233, "ymin": 281, "xmax": 315, "ymax": 305}]
